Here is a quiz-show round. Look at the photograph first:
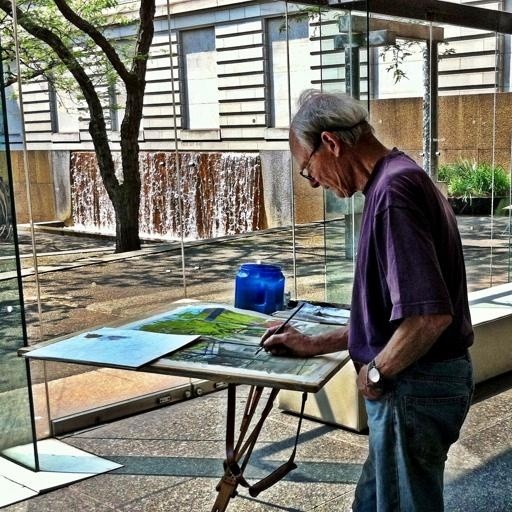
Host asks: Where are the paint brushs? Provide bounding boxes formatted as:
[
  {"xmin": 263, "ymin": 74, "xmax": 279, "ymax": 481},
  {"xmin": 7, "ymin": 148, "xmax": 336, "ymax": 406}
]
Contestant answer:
[{"xmin": 255, "ymin": 302, "xmax": 306, "ymax": 355}]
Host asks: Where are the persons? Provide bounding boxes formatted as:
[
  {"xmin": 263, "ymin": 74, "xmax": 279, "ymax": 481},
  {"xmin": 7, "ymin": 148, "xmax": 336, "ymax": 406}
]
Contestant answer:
[{"xmin": 257, "ymin": 85, "xmax": 477, "ymax": 511}]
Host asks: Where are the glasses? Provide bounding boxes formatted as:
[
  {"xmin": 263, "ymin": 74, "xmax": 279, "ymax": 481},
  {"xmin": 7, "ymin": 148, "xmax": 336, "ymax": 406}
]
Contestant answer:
[{"xmin": 299, "ymin": 140, "xmax": 324, "ymax": 180}]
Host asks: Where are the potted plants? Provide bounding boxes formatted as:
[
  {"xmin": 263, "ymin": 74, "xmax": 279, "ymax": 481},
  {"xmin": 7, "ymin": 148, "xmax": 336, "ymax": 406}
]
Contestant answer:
[{"xmin": 438, "ymin": 156, "xmax": 511, "ymax": 217}]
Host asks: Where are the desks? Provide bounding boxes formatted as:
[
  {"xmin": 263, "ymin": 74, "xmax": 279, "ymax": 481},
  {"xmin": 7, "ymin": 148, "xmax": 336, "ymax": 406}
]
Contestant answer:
[{"xmin": 17, "ymin": 296, "xmax": 350, "ymax": 511}]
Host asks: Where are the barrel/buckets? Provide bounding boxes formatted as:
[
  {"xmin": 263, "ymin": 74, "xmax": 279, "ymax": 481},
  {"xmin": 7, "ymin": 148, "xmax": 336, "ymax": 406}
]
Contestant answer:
[{"xmin": 234, "ymin": 263, "xmax": 285, "ymax": 312}]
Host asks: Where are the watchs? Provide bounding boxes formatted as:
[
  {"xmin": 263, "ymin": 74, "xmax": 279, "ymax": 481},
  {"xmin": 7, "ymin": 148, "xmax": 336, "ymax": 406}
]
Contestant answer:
[{"xmin": 363, "ymin": 360, "xmax": 393, "ymax": 391}]
